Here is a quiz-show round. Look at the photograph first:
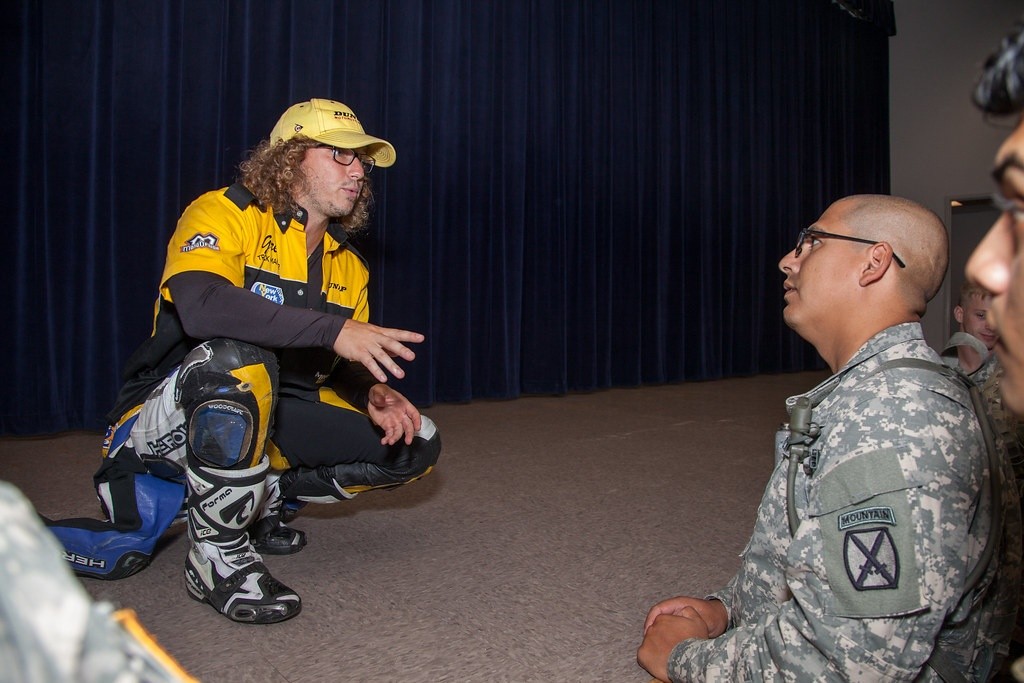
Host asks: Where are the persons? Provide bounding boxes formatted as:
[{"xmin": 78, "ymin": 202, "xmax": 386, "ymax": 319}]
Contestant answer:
[
  {"xmin": 0, "ymin": 483, "xmax": 195, "ymax": 682},
  {"xmin": 951, "ymin": 282, "xmax": 1001, "ymax": 390},
  {"xmin": 93, "ymin": 99, "xmax": 442, "ymax": 623},
  {"xmin": 965, "ymin": 28, "xmax": 1024, "ymax": 413},
  {"xmin": 635, "ymin": 192, "xmax": 1024, "ymax": 683}
]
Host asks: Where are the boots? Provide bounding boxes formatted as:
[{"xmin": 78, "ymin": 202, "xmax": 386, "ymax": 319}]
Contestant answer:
[
  {"xmin": 183, "ymin": 452, "xmax": 302, "ymax": 625},
  {"xmin": 246, "ymin": 463, "xmax": 358, "ymax": 554}
]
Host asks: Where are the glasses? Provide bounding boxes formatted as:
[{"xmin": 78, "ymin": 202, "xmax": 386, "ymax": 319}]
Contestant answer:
[
  {"xmin": 795, "ymin": 226, "xmax": 906, "ymax": 269},
  {"xmin": 317, "ymin": 144, "xmax": 376, "ymax": 174}
]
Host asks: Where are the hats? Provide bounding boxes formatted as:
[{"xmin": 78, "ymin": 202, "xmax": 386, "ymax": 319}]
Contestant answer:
[{"xmin": 270, "ymin": 98, "xmax": 396, "ymax": 168}]
